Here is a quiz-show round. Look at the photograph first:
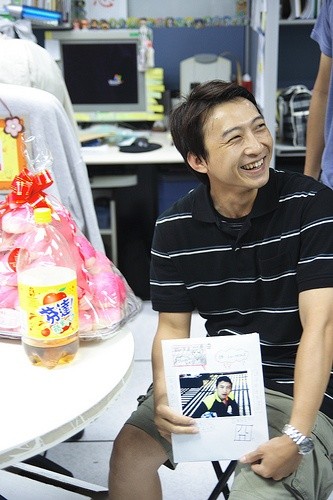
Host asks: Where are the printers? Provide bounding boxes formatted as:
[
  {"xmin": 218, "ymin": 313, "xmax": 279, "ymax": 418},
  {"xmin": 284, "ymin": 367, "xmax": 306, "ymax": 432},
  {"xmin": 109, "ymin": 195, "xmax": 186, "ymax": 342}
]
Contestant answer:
[{"xmin": 180, "ymin": 53, "xmax": 231, "ymax": 104}]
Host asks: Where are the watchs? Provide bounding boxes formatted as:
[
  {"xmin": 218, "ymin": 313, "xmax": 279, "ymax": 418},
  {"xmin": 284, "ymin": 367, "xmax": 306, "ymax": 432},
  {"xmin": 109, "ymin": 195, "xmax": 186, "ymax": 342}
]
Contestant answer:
[{"xmin": 281, "ymin": 423, "xmax": 315, "ymax": 456}]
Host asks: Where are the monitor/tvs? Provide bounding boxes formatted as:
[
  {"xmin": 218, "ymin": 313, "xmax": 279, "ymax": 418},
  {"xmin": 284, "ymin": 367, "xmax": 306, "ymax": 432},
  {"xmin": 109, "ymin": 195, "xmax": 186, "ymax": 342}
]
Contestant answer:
[{"xmin": 45, "ymin": 28, "xmax": 156, "ymax": 141}]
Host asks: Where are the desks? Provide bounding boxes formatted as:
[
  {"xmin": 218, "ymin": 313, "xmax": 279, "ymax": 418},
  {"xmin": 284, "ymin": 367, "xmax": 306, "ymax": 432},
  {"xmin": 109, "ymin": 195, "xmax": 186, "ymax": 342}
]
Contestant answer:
[
  {"xmin": 0, "ymin": 324, "xmax": 134, "ymax": 500},
  {"xmin": 78, "ymin": 126, "xmax": 187, "ymax": 270}
]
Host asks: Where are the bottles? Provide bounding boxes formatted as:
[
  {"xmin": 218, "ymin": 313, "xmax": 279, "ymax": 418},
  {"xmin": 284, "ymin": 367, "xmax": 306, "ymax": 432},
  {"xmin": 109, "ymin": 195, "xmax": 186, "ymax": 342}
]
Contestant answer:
[
  {"xmin": 16, "ymin": 207, "xmax": 80, "ymax": 370},
  {"xmin": 3, "ymin": 4, "xmax": 63, "ymax": 26}
]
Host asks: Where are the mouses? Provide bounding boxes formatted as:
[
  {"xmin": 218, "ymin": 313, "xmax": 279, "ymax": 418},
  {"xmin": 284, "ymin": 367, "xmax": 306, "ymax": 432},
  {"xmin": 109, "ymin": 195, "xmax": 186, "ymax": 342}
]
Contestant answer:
[{"xmin": 134, "ymin": 138, "xmax": 148, "ymax": 148}]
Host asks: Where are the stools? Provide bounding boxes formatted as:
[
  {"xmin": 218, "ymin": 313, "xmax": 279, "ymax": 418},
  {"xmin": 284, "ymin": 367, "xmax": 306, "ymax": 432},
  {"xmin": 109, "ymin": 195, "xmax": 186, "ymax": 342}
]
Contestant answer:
[{"xmin": 90, "ymin": 174, "xmax": 138, "ymax": 267}]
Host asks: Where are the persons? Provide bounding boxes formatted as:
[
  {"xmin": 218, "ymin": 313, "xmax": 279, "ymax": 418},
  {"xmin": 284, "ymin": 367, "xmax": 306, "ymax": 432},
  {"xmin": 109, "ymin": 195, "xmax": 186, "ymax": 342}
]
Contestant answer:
[
  {"xmin": 108, "ymin": 80, "xmax": 333, "ymax": 499},
  {"xmin": 190, "ymin": 376, "xmax": 240, "ymax": 418},
  {"xmin": 304, "ymin": 0, "xmax": 333, "ymax": 190}
]
